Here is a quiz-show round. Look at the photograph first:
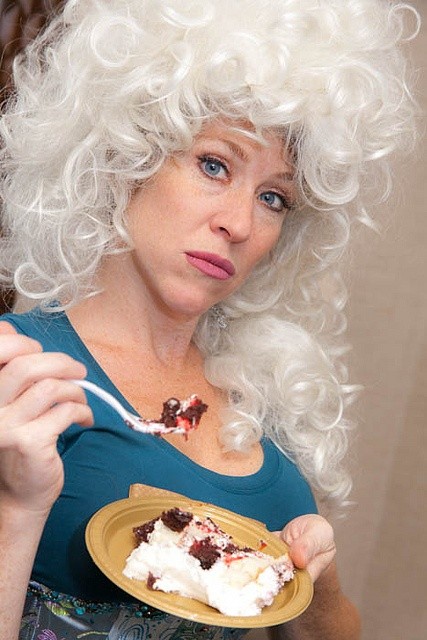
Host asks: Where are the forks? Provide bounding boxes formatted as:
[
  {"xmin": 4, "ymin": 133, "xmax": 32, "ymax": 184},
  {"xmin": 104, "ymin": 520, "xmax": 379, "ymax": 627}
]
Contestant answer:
[{"xmin": 66, "ymin": 377, "xmax": 188, "ymax": 435}]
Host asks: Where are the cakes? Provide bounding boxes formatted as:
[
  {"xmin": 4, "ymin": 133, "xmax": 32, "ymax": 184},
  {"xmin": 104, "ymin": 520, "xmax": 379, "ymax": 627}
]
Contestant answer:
[
  {"xmin": 119, "ymin": 505, "xmax": 295, "ymax": 617},
  {"xmin": 153, "ymin": 395, "xmax": 210, "ymax": 433}
]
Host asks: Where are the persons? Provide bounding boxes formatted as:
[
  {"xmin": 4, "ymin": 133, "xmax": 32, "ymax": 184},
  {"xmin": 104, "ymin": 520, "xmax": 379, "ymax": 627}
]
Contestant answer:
[{"xmin": 0, "ymin": 0, "xmax": 417, "ymax": 640}]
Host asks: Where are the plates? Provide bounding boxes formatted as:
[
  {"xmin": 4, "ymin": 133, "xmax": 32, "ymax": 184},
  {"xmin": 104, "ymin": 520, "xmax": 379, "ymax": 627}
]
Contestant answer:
[{"xmin": 83, "ymin": 495, "xmax": 314, "ymax": 630}]
